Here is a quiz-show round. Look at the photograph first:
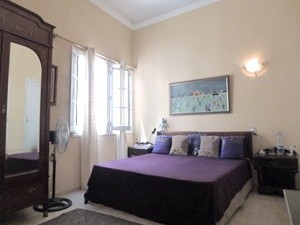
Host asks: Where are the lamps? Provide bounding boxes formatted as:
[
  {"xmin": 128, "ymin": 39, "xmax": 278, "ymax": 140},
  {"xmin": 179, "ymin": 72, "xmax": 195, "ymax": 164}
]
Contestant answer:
[{"xmin": 241, "ymin": 56, "xmax": 266, "ymax": 76}]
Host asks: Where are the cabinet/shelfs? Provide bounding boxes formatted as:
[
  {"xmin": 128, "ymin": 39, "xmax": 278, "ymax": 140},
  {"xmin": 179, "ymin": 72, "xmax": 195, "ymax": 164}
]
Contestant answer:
[{"xmin": 0, "ymin": 0, "xmax": 56, "ymax": 219}]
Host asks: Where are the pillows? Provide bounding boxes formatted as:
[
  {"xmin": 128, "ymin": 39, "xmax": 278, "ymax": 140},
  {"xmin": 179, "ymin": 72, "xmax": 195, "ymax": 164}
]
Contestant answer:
[
  {"xmin": 151, "ymin": 135, "xmax": 173, "ymax": 154},
  {"xmin": 198, "ymin": 135, "xmax": 222, "ymax": 159},
  {"xmin": 168, "ymin": 134, "xmax": 190, "ymax": 157},
  {"xmin": 219, "ymin": 135, "xmax": 248, "ymax": 160}
]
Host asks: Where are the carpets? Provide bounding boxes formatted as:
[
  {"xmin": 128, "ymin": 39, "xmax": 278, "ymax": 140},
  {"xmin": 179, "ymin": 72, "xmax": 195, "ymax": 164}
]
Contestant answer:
[{"xmin": 37, "ymin": 208, "xmax": 143, "ymax": 225}]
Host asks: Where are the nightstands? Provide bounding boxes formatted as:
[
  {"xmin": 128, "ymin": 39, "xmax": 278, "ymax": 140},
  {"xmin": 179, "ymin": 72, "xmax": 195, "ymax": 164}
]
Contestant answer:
[
  {"xmin": 250, "ymin": 148, "xmax": 299, "ymax": 198},
  {"xmin": 128, "ymin": 143, "xmax": 153, "ymax": 158}
]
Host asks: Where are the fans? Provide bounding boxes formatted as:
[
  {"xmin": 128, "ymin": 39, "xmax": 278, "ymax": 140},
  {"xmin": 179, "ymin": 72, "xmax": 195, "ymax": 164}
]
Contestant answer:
[{"xmin": 32, "ymin": 116, "xmax": 73, "ymax": 213}]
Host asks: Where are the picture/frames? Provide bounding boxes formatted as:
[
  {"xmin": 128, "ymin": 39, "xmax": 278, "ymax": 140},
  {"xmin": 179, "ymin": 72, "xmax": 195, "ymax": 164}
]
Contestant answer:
[
  {"xmin": 168, "ymin": 74, "xmax": 231, "ymax": 116},
  {"xmin": 49, "ymin": 65, "xmax": 59, "ymax": 106}
]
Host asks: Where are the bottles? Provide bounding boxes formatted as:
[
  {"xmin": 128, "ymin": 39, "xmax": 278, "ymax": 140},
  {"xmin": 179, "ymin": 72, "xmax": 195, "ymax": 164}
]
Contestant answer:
[{"xmin": 276, "ymin": 131, "xmax": 285, "ymax": 156}]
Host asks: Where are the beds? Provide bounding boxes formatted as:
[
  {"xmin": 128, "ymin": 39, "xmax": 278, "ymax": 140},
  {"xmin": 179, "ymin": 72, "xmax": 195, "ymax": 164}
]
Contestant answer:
[{"xmin": 84, "ymin": 131, "xmax": 256, "ymax": 225}]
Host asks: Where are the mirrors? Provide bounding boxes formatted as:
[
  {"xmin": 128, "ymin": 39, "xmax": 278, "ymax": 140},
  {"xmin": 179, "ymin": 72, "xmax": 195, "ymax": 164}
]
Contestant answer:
[{"xmin": 4, "ymin": 40, "xmax": 42, "ymax": 161}]
